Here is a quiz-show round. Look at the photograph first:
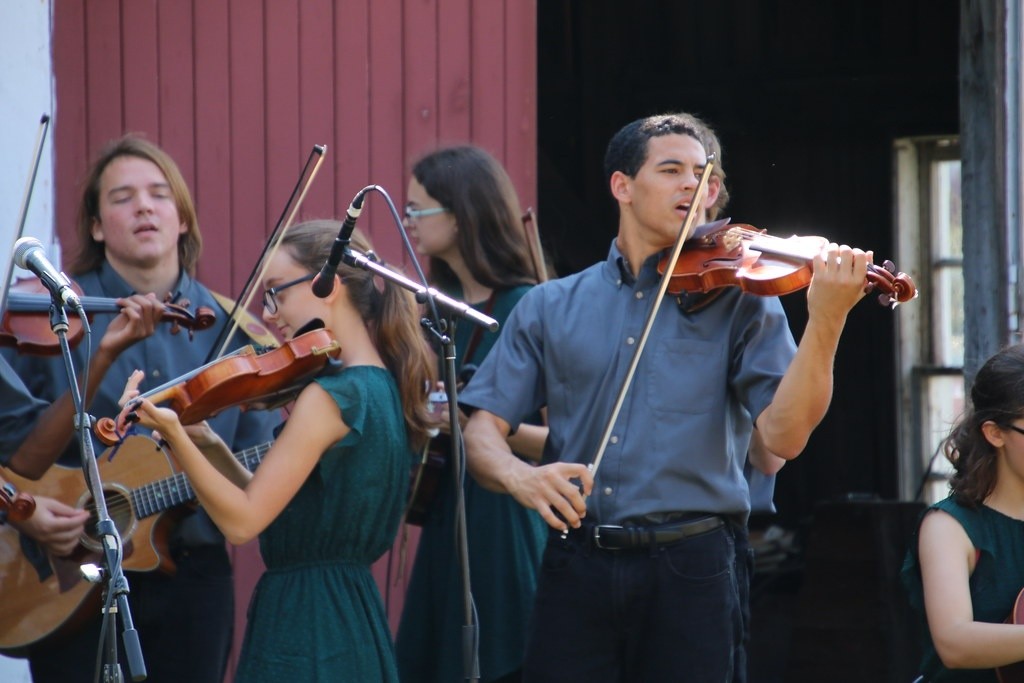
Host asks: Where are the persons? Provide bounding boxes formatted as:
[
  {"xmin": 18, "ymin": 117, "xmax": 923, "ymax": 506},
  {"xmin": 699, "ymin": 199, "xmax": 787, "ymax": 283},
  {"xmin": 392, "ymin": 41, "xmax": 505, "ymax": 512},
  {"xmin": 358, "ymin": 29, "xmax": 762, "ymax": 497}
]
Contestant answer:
[
  {"xmin": 913, "ymin": 343, "xmax": 1024, "ymax": 683},
  {"xmin": 454, "ymin": 117, "xmax": 870, "ymax": 683},
  {"xmin": 0, "ymin": 132, "xmax": 280, "ymax": 683},
  {"xmin": 118, "ymin": 221, "xmax": 441, "ymax": 683},
  {"xmin": 404, "ymin": 147, "xmax": 552, "ymax": 683}
]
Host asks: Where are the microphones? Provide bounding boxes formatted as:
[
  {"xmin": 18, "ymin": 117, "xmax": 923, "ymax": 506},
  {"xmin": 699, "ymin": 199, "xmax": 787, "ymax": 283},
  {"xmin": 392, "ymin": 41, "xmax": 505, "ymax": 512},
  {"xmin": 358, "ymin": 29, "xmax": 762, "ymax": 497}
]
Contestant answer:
[
  {"xmin": 13, "ymin": 237, "xmax": 83, "ymax": 310},
  {"xmin": 312, "ymin": 190, "xmax": 366, "ymax": 298}
]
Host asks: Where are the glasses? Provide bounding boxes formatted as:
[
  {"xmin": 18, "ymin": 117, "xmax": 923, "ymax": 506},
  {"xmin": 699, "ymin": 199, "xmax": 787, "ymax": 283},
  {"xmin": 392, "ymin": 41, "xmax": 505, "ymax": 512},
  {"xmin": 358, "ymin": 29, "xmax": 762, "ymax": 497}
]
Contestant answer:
[
  {"xmin": 404, "ymin": 206, "xmax": 451, "ymax": 223},
  {"xmin": 262, "ymin": 272, "xmax": 348, "ymax": 315}
]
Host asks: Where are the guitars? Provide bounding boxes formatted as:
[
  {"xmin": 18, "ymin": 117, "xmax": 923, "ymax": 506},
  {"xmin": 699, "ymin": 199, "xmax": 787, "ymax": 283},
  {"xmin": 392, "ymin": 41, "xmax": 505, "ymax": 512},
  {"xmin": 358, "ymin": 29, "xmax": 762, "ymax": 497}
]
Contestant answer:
[
  {"xmin": 400, "ymin": 393, "xmax": 460, "ymax": 528},
  {"xmin": 0, "ymin": 432, "xmax": 285, "ymax": 659},
  {"xmin": 994, "ymin": 587, "xmax": 1024, "ymax": 683}
]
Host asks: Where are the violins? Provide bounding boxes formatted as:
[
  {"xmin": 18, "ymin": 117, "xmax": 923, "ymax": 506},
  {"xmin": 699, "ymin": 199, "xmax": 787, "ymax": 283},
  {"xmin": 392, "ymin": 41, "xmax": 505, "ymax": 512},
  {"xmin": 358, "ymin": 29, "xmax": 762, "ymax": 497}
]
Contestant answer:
[
  {"xmin": 3, "ymin": 273, "xmax": 217, "ymax": 356},
  {"xmin": 656, "ymin": 219, "xmax": 920, "ymax": 304},
  {"xmin": 91, "ymin": 324, "xmax": 342, "ymax": 449}
]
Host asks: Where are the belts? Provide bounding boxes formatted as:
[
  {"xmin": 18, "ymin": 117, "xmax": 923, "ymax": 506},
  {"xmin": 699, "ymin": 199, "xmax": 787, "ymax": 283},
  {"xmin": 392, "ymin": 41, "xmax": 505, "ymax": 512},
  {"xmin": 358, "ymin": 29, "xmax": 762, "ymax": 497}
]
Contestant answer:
[{"xmin": 548, "ymin": 516, "xmax": 723, "ymax": 552}]
